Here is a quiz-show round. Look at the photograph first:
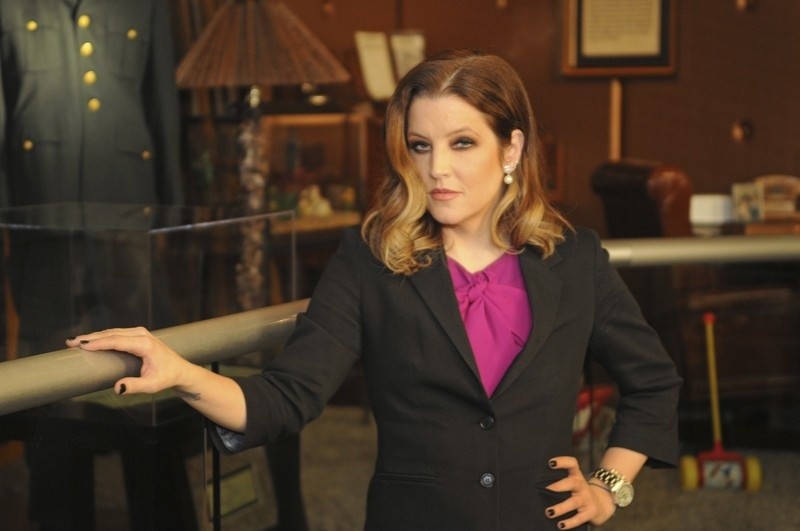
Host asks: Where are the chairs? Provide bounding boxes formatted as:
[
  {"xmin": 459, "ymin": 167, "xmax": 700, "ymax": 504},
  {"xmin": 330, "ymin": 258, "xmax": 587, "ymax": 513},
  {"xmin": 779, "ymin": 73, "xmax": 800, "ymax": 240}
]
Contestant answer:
[{"xmin": 584, "ymin": 158, "xmax": 800, "ymax": 451}]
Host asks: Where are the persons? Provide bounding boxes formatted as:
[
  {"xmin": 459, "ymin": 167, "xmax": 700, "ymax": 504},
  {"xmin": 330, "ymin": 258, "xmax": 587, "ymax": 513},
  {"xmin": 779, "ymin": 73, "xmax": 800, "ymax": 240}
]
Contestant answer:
[{"xmin": 65, "ymin": 48, "xmax": 682, "ymax": 531}]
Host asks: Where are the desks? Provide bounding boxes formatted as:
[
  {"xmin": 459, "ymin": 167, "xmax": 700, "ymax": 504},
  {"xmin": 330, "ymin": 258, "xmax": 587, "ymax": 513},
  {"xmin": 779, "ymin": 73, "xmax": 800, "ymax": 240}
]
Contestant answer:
[
  {"xmin": 0, "ymin": 200, "xmax": 363, "ymax": 364},
  {"xmin": 23, "ymin": 366, "xmax": 312, "ymax": 531}
]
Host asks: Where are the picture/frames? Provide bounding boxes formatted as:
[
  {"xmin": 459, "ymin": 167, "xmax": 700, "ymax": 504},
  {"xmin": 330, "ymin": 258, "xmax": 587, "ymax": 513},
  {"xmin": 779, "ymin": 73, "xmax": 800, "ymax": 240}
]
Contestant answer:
[{"xmin": 564, "ymin": 0, "xmax": 679, "ymax": 77}]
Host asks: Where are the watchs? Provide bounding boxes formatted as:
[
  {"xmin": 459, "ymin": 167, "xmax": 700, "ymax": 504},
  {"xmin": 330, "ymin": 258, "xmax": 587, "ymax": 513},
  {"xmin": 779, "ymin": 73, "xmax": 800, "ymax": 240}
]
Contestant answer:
[{"xmin": 588, "ymin": 468, "xmax": 635, "ymax": 509}]
